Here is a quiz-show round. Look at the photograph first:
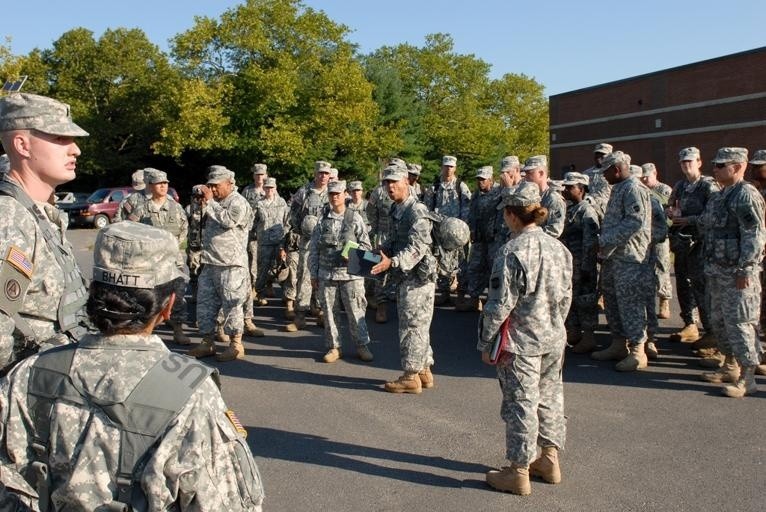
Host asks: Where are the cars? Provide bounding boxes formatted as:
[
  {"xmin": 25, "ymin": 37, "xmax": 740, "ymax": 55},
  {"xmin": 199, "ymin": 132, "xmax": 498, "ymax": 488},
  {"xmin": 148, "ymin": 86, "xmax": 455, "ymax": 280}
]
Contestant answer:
[{"xmin": 52, "ymin": 191, "xmax": 90, "ymax": 206}]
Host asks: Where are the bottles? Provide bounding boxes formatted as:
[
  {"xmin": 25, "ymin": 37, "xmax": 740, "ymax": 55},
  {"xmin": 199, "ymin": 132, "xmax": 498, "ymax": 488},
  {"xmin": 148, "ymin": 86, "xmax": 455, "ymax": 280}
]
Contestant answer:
[{"xmin": 673, "ymin": 198, "xmax": 682, "ymax": 218}]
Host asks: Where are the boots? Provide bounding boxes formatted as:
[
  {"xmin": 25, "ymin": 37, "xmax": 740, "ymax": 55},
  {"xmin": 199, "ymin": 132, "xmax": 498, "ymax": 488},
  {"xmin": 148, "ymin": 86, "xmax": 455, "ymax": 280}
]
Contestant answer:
[
  {"xmin": 487, "ymin": 460, "xmax": 531, "ymax": 496},
  {"xmin": 171, "ymin": 283, "xmax": 389, "ymax": 362},
  {"xmin": 434, "ymin": 273, "xmax": 483, "ymax": 312},
  {"xmin": 564, "ymin": 295, "xmax": 671, "ymax": 370},
  {"xmin": 530, "ymin": 447, "xmax": 561, "ymax": 483},
  {"xmin": 384, "ymin": 367, "xmax": 433, "ymax": 393},
  {"xmin": 674, "ymin": 324, "xmax": 766, "ymax": 398}
]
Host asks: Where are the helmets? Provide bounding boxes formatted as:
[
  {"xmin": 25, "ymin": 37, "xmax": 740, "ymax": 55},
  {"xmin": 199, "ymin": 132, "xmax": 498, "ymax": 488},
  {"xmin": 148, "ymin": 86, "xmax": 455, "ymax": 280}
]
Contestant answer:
[
  {"xmin": 301, "ymin": 215, "xmax": 319, "ymax": 235},
  {"xmin": 439, "ymin": 216, "xmax": 470, "ymax": 250},
  {"xmin": 268, "ymin": 267, "xmax": 289, "ymax": 282}
]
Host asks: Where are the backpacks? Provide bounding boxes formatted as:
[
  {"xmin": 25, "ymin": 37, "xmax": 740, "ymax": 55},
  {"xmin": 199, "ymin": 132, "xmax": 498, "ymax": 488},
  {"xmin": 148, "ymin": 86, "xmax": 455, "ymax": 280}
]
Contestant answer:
[{"xmin": 409, "ymin": 209, "xmax": 467, "ymax": 278}]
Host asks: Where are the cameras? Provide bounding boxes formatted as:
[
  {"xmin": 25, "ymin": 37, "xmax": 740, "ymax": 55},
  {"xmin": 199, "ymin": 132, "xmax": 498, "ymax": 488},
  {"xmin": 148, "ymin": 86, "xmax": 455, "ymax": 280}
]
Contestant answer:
[{"xmin": 192, "ymin": 188, "xmax": 202, "ymax": 195}]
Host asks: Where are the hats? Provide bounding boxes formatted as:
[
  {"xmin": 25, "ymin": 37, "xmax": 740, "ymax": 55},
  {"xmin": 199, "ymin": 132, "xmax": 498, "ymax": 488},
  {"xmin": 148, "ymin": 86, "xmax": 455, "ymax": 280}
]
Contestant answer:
[
  {"xmin": 132, "ymin": 167, "xmax": 169, "ymax": 191},
  {"xmin": 389, "ymin": 158, "xmax": 405, "ymax": 165},
  {"xmin": 0, "ymin": 92, "xmax": 91, "ymax": 138},
  {"xmin": 263, "ymin": 178, "xmax": 276, "ymax": 189},
  {"xmin": 562, "ymin": 172, "xmax": 590, "ymax": 186},
  {"xmin": 251, "ymin": 164, "xmax": 269, "ymax": 175},
  {"xmin": 443, "ymin": 155, "xmax": 457, "ymax": 166},
  {"xmin": 383, "ymin": 165, "xmax": 409, "ymax": 180},
  {"xmin": 193, "ymin": 166, "xmax": 236, "ymax": 196},
  {"xmin": 500, "ymin": 156, "xmax": 519, "ymax": 172},
  {"xmin": 523, "ymin": 155, "xmax": 547, "ymax": 171},
  {"xmin": 93, "ymin": 219, "xmax": 191, "ymax": 289},
  {"xmin": 496, "ymin": 182, "xmax": 542, "ymax": 211},
  {"xmin": 749, "ymin": 149, "xmax": 766, "ymax": 165},
  {"xmin": 408, "ymin": 164, "xmax": 422, "ymax": 175},
  {"xmin": 642, "ymin": 163, "xmax": 656, "ymax": 176},
  {"xmin": 599, "ymin": 151, "xmax": 631, "ymax": 172},
  {"xmin": 678, "ymin": 147, "xmax": 701, "ymax": 161},
  {"xmin": 316, "ymin": 161, "xmax": 363, "ymax": 194},
  {"xmin": 594, "ymin": 143, "xmax": 613, "ymax": 154},
  {"xmin": 475, "ymin": 166, "xmax": 494, "ymax": 179},
  {"xmin": 630, "ymin": 165, "xmax": 642, "ymax": 177},
  {"xmin": 710, "ymin": 147, "xmax": 749, "ymax": 164}
]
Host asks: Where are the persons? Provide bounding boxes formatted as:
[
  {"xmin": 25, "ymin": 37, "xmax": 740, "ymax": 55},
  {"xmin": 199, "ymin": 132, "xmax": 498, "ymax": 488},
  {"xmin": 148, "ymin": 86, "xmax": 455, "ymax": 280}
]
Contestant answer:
[
  {"xmin": 0, "ymin": 219, "xmax": 265, "ymax": 511},
  {"xmin": 471, "ymin": 143, "xmax": 766, "ymax": 399},
  {"xmin": 117, "ymin": 166, "xmax": 288, "ymax": 361},
  {"xmin": 284, "ymin": 156, "xmax": 472, "ymax": 395},
  {"xmin": 477, "ymin": 182, "xmax": 573, "ymax": 495},
  {"xmin": 1, "ymin": 93, "xmax": 90, "ymax": 368}
]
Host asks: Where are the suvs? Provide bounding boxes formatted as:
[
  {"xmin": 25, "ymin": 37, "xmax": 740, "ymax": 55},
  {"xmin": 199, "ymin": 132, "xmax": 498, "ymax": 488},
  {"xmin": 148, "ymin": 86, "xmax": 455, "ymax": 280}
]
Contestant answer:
[{"xmin": 54, "ymin": 185, "xmax": 179, "ymax": 229}]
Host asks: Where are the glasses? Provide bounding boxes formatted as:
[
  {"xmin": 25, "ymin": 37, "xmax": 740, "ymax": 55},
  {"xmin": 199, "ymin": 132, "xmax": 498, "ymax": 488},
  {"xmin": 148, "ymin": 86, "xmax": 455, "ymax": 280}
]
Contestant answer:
[{"xmin": 712, "ymin": 163, "xmax": 735, "ymax": 168}]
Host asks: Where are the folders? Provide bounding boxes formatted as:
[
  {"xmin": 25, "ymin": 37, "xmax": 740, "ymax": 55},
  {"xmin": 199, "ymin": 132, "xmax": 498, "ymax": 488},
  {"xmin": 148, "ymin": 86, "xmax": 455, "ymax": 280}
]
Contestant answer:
[
  {"xmin": 480, "ymin": 316, "xmax": 510, "ymax": 364},
  {"xmin": 347, "ymin": 248, "xmax": 388, "ymax": 281}
]
